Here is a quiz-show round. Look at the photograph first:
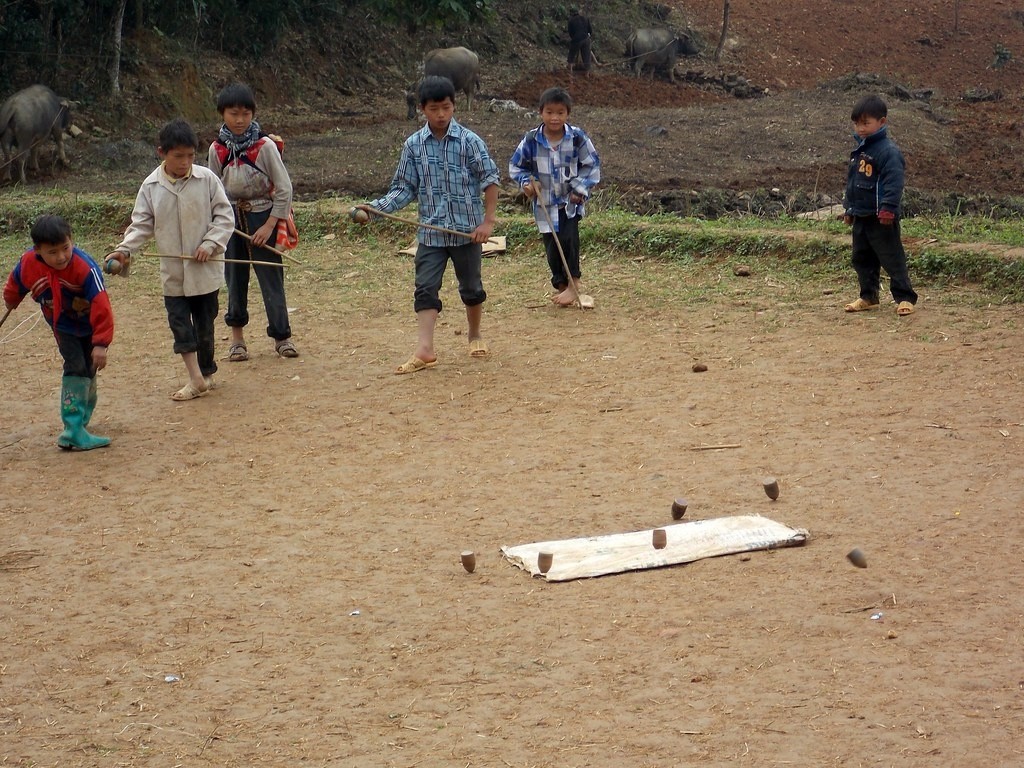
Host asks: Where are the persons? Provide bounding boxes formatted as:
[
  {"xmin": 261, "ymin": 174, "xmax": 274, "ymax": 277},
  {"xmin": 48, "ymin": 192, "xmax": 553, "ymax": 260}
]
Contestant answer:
[
  {"xmin": 837, "ymin": 94, "xmax": 919, "ymax": 316},
  {"xmin": 509, "ymin": 85, "xmax": 604, "ymax": 308},
  {"xmin": 103, "ymin": 119, "xmax": 236, "ymax": 401},
  {"xmin": 206, "ymin": 81, "xmax": 301, "ymax": 362},
  {"xmin": 565, "ymin": 7, "xmax": 597, "ymax": 80},
  {"xmin": 4, "ymin": 213, "xmax": 116, "ymax": 453},
  {"xmin": 348, "ymin": 73, "xmax": 501, "ymax": 376}
]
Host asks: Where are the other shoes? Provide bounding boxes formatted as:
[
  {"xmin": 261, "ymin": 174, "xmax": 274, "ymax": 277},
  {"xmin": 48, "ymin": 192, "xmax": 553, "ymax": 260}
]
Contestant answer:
[
  {"xmin": 82, "ymin": 376, "xmax": 112, "ymax": 447},
  {"xmin": 57, "ymin": 376, "xmax": 97, "ymax": 451}
]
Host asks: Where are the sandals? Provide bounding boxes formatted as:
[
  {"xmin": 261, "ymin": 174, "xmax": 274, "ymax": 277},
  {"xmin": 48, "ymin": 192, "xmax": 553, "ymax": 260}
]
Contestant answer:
[
  {"xmin": 229, "ymin": 343, "xmax": 249, "ymax": 362},
  {"xmin": 275, "ymin": 341, "xmax": 299, "ymax": 356}
]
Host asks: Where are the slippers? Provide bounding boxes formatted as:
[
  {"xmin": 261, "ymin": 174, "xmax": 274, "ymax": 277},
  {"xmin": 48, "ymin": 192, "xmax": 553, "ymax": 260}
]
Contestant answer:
[
  {"xmin": 845, "ymin": 297, "xmax": 880, "ymax": 312},
  {"xmin": 469, "ymin": 340, "xmax": 490, "ymax": 357},
  {"xmin": 394, "ymin": 355, "xmax": 438, "ymax": 374},
  {"xmin": 203, "ymin": 374, "xmax": 216, "ymax": 390},
  {"xmin": 897, "ymin": 301, "xmax": 915, "ymax": 315},
  {"xmin": 171, "ymin": 384, "xmax": 210, "ymax": 401}
]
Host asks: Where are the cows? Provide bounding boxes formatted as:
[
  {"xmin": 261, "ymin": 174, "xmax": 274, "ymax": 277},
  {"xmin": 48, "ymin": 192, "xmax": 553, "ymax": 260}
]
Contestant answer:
[
  {"xmin": 627, "ymin": 28, "xmax": 700, "ymax": 85},
  {"xmin": 424, "ymin": 46, "xmax": 480, "ymax": 111},
  {"xmin": 0, "ymin": 84, "xmax": 81, "ymax": 186}
]
